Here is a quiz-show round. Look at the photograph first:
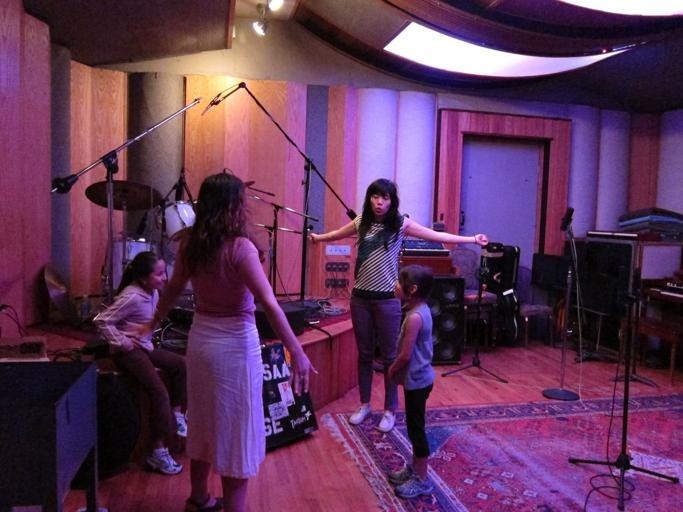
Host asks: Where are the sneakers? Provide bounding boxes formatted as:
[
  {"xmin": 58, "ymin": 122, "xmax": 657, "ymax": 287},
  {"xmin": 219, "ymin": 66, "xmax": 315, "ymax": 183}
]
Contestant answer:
[
  {"xmin": 349, "ymin": 403, "xmax": 372, "ymax": 424},
  {"xmin": 147, "ymin": 447, "xmax": 184, "ymax": 475},
  {"xmin": 174, "ymin": 412, "xmax": 188, "ymax": 438},
  {"xmin": 394, "ymin": 474, "xmax": 436, "ymax": 499},
  {"xmin": 388, "ymin": 463, "xmax": 413, "ymax": 484},
  {"xmin": 378, "ymin": 410, "xmax": 396, "ymax": 431}
]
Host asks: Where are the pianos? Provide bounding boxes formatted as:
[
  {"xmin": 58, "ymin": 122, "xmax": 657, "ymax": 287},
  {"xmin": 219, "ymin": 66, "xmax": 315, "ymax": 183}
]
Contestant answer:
[
  {"xmin": 637, "ymin": 270, "xmax": 683, "ymax": 369},
  {"xmin": 399, "ymin": 238, "xmax": 451, "ymax": 257}
]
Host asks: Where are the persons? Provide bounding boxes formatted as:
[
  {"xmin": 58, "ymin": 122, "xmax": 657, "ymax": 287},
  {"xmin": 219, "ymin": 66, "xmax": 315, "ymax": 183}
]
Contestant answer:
[
  {"xmin": 307, "ymin": 178, "xmax": 488, "ymax": 432},
  {"xmin": 135, "ymin": 173, "xmax": 319, "ymax": 511},
  {"xmin": 93, "ymin": 251, "xmax": 187, "ymax": 475},
  {"xmin": 271, "ymin": 346, "xmax": 284, "ymax": 369},
  {"xmin": 281, "ymin": 383, "xmax": 295, "ymax": 406},
  {"xmin": 386, "ymin": 265, "xmax": 436, "ymax": 497}
]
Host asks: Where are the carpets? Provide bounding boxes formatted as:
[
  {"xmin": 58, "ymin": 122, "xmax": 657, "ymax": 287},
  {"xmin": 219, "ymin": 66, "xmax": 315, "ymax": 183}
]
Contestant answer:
[{"xmin": 321, "ymin": 393, "xmax": 683, "ymax": 512}]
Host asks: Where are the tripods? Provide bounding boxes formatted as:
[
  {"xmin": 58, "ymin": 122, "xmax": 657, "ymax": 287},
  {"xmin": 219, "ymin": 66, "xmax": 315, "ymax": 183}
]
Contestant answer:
[
  {"xmin": 441, "ymin": 247, "xmax": 507, "ymax": 383},
  {"xmin": 567, "ymin": 244, "xmax": 680, "ymax": 508},
  {"xmin": 568, "ymin": 296, "xmax": 676, "ymax": 511}
]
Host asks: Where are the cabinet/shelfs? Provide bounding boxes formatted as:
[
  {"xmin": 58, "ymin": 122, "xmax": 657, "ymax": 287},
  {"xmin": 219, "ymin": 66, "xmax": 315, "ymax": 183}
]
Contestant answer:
[
  {"xmin": 561, "ymin": 236, "xmax": 683, "ymax": 363},
  {"xmin": 0, "ymin": 362, "xmax": 99, "ymax": 512}
]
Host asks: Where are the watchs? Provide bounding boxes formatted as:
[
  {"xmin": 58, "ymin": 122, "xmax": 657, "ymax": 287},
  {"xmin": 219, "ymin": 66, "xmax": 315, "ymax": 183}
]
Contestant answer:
[{"xmin": 153, "ymin": 313, "xmax": 169, "ymax": 323}]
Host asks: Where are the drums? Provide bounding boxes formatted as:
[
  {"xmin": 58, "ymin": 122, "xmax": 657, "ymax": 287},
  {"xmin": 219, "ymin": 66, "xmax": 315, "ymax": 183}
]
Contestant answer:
[
  {"xmin": 152, "ymin": 201, "xmax": 196, "ymax": 257},
  {"xmin": 103, "ymin": 236, "xmax": 158, "ymax": 291}
]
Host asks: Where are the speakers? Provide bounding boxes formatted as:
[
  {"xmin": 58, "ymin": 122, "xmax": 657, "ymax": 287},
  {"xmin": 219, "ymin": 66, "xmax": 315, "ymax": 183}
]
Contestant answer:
[{"xmin": 425, "ymin": 276, "xmax": 464, "ymax": 363}]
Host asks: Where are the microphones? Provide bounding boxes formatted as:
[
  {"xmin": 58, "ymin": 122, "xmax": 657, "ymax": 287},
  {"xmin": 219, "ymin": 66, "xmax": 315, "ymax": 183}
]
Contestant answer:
[
  {"xmin": 560, "ymin": 207, "xmax": 574, "ymax": 233},
  {"xmin": 84, "ymin": 339, "xmax": 108, "ymax": 355}
]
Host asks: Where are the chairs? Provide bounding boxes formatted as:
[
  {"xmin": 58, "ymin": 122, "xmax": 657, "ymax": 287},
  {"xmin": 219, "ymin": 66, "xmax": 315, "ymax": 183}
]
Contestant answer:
[
  {"xmin": 448, "ymin": 247, "xmax": 497, "ymax": 349},
  {"xmin": 506, "ymin": 267, "xmax": 552, "ymax": 349}
]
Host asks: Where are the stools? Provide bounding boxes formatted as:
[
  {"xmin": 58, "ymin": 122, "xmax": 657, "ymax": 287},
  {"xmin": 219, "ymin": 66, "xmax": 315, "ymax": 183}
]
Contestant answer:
[{"xmin": 621, "ymin": 317, "xmax": 683, "ymax": 387}]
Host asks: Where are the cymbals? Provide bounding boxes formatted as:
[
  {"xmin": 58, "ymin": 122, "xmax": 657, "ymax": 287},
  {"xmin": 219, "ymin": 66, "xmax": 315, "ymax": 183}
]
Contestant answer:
[
  {"xmin": 43, "ymin": 264, "xmax": 81, "ymax": 328},
  {"xmin": 85, "ymin": 179, "xmax": 168, "ymax": 212}
]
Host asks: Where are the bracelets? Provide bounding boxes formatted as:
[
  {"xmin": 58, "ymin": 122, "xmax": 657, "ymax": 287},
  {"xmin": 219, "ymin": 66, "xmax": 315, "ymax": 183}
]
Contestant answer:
[{"xmin": 474, "ymin": 236, "xmax": 477, "ymax": 244}]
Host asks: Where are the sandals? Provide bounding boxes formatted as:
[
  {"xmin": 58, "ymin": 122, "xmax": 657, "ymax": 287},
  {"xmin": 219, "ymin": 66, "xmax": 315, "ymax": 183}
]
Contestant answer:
[{"xmin": 185, "ymin": 492, "xmax": 224, "ymax": 512}]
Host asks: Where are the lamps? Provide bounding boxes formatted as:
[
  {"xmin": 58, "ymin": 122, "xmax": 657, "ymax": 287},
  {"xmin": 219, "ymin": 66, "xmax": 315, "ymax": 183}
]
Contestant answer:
[
  {"xmin": 252, "ymin": 2, "xmax": 267, "ymax": 35},
  {"xmin": 268, "ymin": 0, "xmax": 283, "ymax": 12}
]
